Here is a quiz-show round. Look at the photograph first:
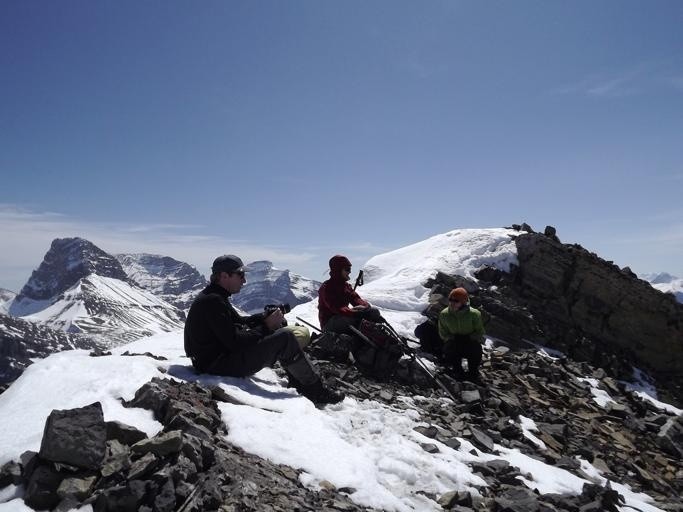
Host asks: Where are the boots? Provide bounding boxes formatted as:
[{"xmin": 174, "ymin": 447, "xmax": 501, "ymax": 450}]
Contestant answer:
[{"xmin": 294, "ymin": 374, "xmax": 345, "ymax": 405}]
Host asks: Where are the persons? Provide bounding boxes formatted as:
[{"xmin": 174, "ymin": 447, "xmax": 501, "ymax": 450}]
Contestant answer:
[
  {"xmin": 438, "ymin": 288, "xmax": 488, "ymax": 388},
  {"xmin": 318, "ymin": 255, "xmax": 387, "ymax": 381},
  {"xmin": 184, "ymin": 255, "xmax": 346, "ymax": 408}
]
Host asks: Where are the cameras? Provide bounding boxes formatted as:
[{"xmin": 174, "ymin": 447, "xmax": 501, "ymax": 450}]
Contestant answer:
[{"xmin": 264, "ymin": 304, "xmax": 290, "ymax": 313}]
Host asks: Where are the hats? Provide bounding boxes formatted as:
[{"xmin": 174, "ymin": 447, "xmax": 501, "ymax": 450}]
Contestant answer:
[
  {"xmin": 449, "ymin": 287, "xmax": 468, "ymax": 305},
  {"xmin": 213, "ymin": 255, "xmax": 256, "ymax": 273},
  {"xmin": 329, "ymin": 256, "xmax": 352, "ymax": 268}
]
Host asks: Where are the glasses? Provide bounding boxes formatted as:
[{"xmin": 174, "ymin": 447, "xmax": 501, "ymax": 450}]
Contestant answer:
[
  {"xmin": 232, "ymin": 272, "xmax": 244, "ymax": 278},
  {"xmin": 345, "ymin": 267, "xmax": 351, "ymax": 273}
]
